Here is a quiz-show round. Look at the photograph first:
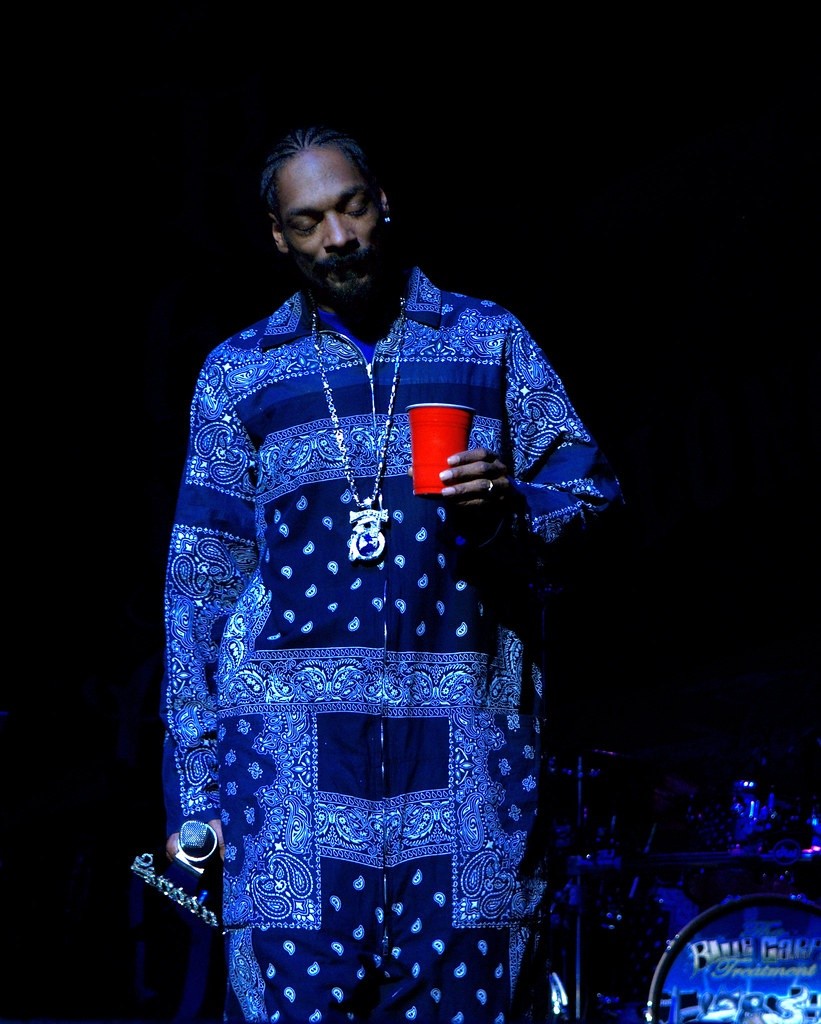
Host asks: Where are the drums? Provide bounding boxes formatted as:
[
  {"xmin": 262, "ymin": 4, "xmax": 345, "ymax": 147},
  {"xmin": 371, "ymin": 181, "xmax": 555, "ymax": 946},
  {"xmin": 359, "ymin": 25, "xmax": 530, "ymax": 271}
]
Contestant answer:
[{"xmin": 638, "ymin": 888, "xmax": 821, "ymax": 1023}]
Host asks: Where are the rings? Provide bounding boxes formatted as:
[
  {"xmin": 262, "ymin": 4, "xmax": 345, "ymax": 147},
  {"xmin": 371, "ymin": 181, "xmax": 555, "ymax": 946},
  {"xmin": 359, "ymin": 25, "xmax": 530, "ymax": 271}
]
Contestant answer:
[{"xmin": 488, "ymin": 480, "xmax": 493, "ymax": 492}]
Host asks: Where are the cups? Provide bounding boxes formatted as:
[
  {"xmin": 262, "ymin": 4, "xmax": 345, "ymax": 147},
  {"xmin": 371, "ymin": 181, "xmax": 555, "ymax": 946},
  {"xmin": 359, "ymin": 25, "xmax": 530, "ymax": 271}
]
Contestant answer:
[{"xmin": 406, "ymin": 402, "xmax": 476, "ymax": 496}]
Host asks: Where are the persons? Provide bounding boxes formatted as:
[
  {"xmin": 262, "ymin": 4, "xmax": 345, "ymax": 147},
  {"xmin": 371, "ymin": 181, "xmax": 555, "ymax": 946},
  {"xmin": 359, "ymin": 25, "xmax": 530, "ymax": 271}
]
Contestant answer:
[{"xmin": 160, "ymin": 126, "xmax": 624, "ymax": 1024}]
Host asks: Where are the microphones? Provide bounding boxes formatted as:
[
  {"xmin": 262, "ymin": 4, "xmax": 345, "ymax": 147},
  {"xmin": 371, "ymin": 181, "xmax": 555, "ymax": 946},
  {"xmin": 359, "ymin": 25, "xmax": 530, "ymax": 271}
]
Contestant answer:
[{"xmin": 177, "ymin": 819, "xmax": 220, "ymax": 868}]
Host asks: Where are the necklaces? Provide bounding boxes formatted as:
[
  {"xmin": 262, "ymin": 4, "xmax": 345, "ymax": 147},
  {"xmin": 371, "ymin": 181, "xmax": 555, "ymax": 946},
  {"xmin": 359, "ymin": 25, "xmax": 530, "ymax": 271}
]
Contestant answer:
[{"xmin": 308, "ymin": 289, "xmax": 405, "ymax": 562}]
